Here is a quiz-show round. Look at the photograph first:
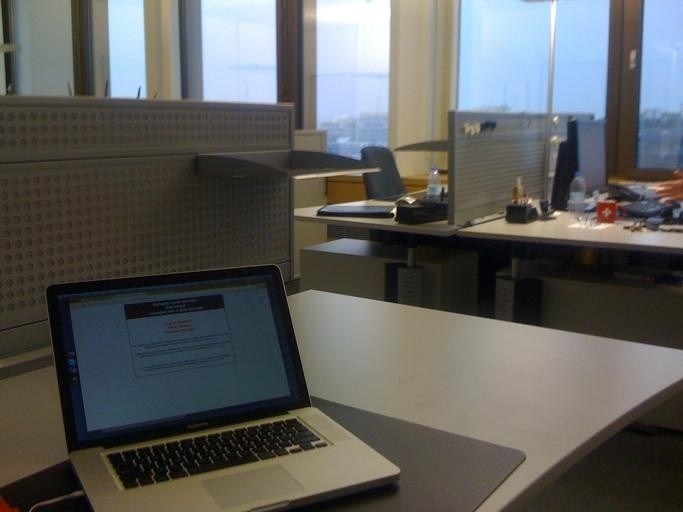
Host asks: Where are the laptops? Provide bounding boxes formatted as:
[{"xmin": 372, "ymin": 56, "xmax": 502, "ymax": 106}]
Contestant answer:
[{"xmin": 46, "ymin": 263, "xmax": 401, "ymax": 511}]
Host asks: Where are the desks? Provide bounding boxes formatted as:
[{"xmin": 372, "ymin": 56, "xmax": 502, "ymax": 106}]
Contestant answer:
[
  {"xmin": 293, "ymin": 182, "xmax": 474, "ymax": 304},
  {"xmin": 1, "ymin": 289, "xmax": 683, "ymax": 512},
  {"xmin": 456, "ymin": 194, "xmax": 683, "ymax": 326}
]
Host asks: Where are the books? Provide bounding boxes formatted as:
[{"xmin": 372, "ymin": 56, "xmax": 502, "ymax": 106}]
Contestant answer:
[{"xmin": 316, "ymin": 202, "xmax": 397, "ymax": 219}]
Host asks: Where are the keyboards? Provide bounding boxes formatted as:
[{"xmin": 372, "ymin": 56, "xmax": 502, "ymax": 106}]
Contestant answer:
[{"xmin": 622, "ymin": 199, "xmax": 674, "ymax": 218}]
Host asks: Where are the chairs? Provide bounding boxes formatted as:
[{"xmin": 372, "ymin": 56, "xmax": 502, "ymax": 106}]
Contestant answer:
[{"xmin": 361, "ymin": 146, "xmax": 410, "ymax": 244}]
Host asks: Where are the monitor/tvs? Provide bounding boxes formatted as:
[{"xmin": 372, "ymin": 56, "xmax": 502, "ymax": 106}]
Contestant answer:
[{"xmin": 551, "ymin": 117, "xmax": 609, "ymax": 211}]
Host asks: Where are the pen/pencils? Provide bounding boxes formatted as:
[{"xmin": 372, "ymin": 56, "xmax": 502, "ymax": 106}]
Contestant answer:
[{"xmin": 512, "ymin": 176, "xmax": 529, "ymax": 207}]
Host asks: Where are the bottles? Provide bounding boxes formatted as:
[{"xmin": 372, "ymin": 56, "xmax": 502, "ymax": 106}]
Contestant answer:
[
  {"xmin": 569, "ymin": 171, "xmax": 585, "ymax": 224},
  {"xmin": 426, "ymin": 166, "xmax": 442, "ymax": 198}
]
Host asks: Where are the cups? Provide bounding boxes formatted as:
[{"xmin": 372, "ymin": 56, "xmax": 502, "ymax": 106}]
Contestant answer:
[{"xmin": 597, "ymin": 201, "xmax": 624, "ymax": 223}]
